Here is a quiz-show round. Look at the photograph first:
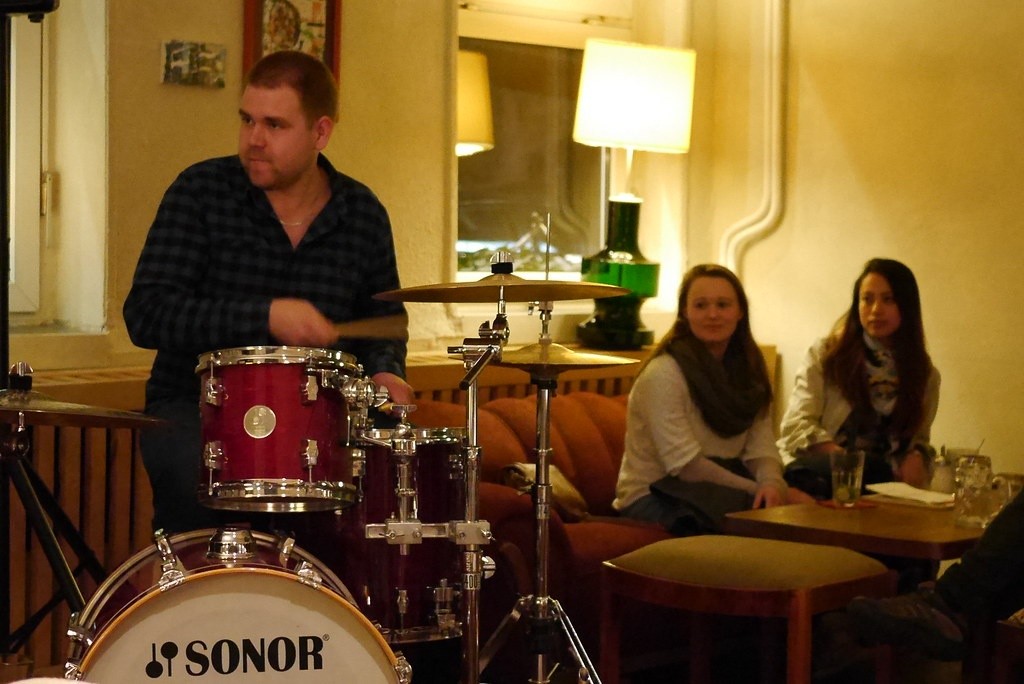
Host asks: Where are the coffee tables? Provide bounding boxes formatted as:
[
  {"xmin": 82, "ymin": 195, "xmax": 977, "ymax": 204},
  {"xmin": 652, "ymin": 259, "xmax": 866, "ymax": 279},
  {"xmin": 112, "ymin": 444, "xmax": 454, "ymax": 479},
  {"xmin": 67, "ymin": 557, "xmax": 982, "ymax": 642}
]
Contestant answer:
[{"xmin": 723, "ymin": 473, "xmax": 1024, "ymax": 684}]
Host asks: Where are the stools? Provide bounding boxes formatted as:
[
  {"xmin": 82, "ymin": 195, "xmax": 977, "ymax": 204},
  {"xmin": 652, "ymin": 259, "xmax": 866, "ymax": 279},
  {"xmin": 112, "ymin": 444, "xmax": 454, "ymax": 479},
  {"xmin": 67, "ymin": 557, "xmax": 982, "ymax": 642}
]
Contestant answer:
[{"xmin": 597, "ymin": 533, "xmax": 899, "ymax": 684}]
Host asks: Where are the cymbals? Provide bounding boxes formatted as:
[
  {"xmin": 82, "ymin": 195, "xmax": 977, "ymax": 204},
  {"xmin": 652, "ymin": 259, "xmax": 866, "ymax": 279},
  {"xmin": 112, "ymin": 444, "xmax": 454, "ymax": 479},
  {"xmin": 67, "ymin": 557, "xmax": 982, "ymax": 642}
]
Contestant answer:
[
  {"xmin": 448, "ymin": 338, "xmax": 644, "ymax": 379},
  {"xmin": 368, "ymin": 271, "xmax": 633, "ymax": 304},
  {"xmin": 0, "ymin": 374, "xmax": 169, "ymax": 430}
]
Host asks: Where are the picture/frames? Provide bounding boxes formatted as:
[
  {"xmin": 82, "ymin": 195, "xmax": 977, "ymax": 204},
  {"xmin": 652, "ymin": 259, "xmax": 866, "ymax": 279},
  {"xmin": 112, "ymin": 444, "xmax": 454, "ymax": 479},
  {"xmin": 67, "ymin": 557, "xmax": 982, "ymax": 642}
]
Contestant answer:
[{"xmin": 243, "ymin": 0, "xmax": 343, "ymax": 126}]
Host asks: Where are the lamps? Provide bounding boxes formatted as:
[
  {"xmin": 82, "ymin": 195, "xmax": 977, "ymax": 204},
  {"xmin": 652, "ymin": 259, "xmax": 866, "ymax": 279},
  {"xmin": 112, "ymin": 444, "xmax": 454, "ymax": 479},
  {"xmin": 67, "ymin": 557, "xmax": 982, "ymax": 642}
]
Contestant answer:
[
  {"xmin": 455, "ymin": 50, "xmax": 494, "ymax": 157},
  {"xmin": 572, "ymin": 38, "xmax": 697, "ymax": 348}
]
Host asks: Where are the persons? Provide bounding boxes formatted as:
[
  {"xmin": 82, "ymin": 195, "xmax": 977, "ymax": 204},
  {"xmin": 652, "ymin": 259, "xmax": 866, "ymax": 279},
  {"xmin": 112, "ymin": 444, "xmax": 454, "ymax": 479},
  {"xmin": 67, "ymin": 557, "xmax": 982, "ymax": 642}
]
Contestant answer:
[
  {"xmin": 124, "ymin": 51, "xmax": 416, "ymax": 574},
  {"xmin": 611, "ymin": 264, "xmax": 818, "ymax": 532},
  {"xmin": 774, "ymin": 257, "xmax": 942, "ymax": 597},
  {"xmin": 847, "ymin": 482, "xmax": 1024, "ymax": 661}
]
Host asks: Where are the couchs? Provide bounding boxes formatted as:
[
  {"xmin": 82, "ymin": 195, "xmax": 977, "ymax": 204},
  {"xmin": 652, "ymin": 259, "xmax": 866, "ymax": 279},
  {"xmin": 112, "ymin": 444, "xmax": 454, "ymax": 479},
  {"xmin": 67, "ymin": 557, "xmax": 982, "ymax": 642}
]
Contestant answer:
[{"xmin": 408, "ymin": 394, "xmax": 765, "ymax": 684}]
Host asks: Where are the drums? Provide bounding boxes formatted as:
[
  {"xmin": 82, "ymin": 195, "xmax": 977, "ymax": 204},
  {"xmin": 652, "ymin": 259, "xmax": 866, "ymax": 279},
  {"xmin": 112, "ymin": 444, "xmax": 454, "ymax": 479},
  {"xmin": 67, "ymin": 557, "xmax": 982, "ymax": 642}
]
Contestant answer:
[
  {"xmin": 193, "ymin": 343, "xmax": 391, "ymax": 513},
  {"xmin": 277, "ymin": 426, "xmax": 471, "ymax": 647},
  {"xmin": 64, "ymin": 525, "xmax": 413, "ymax": 684}
]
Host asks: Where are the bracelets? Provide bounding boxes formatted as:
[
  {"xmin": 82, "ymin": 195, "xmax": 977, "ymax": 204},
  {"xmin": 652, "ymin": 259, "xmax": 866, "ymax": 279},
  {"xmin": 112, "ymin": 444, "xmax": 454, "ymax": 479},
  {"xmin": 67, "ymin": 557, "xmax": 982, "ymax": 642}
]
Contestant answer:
[{"xmin": 906, "ymin": 448, "xmax": 924, "ymax": 458}]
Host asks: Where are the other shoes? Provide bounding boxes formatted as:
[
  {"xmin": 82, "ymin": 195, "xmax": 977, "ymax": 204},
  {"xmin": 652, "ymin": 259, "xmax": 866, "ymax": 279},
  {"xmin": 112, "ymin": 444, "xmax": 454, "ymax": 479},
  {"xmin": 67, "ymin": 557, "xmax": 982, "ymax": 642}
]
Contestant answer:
[
  {"xmin": 840, "ymin": 588, "xmax": 969, "ymax": 658},
  {"xmin": 995, "ymin": 608, "xmax": 1024, "ymax": 629}
]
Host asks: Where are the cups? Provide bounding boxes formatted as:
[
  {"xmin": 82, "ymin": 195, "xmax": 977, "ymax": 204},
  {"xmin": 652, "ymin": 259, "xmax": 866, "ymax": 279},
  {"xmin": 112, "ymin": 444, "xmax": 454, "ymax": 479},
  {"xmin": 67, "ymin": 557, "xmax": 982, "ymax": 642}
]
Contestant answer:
[
  {"xmin": 828, "ymin": 449, "xmax": 864, "ymax": 510},
  {"xmin": 952, "ymin": 455, "xmax": 1012, "ymax": 530}
]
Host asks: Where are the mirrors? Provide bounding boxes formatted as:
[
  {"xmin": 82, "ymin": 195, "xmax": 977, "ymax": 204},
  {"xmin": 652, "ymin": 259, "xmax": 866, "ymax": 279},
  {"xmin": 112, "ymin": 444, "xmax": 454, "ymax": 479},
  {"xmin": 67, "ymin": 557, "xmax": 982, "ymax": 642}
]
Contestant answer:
[{"xmin": 457, "ymin": 8, "xmax": 611, "ymax": 281}]
